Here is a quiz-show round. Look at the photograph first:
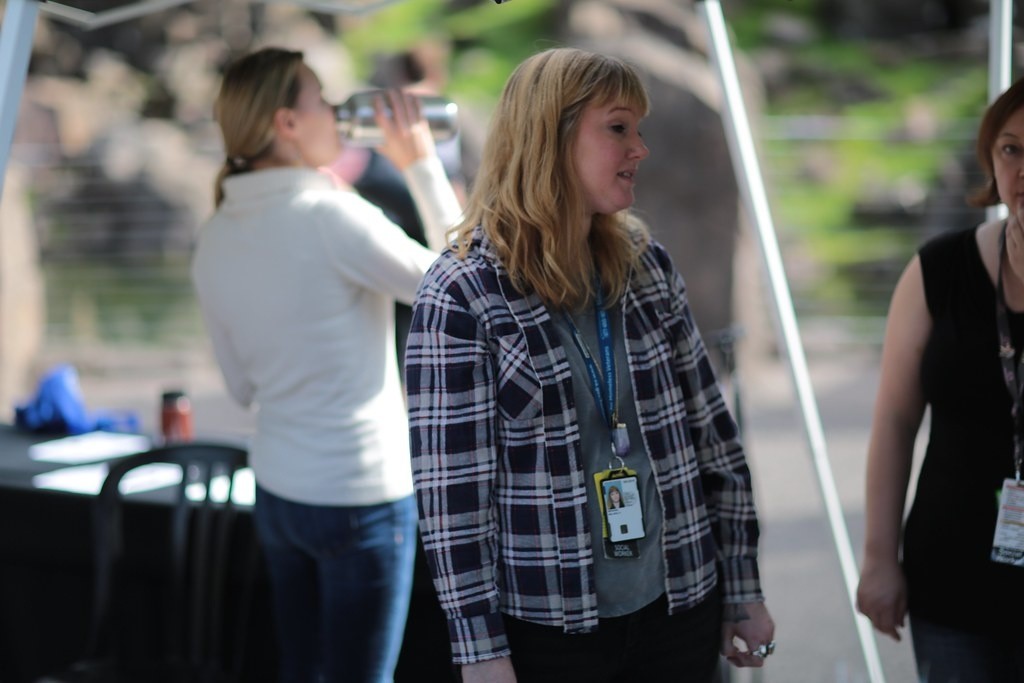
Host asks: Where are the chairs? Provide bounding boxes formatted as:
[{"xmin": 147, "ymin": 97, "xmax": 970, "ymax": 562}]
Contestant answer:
[{"xmin": 82, "ymin": 442, "xmax": 270, "ymax": 682}]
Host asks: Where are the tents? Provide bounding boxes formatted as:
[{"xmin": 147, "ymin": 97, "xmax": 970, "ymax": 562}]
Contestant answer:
[{"xmin": 0, "ymin": 0, "xmax": 1009, "ymax": 683}]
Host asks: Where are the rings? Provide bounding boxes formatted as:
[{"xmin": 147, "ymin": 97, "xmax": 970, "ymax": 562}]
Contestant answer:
[
  {"xmin": 751, "ymin": 645, "xmax": 768, "ymax": 658},
  {"xmin": 768, "ymin": 642, "xmax": 776, "ymax": 654}
]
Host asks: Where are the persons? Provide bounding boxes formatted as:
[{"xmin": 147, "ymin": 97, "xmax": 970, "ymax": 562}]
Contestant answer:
[
  {"xmin": 358, "ymin": 45, "xmax": 476, "ymax": 365},
  {"xmin": 608, "ymin": 486, "xmax": 624, "ymax": 509},
  {"xmin": 405, "ymin": 47, "xmax": 776, "ymax": 683},
  {"xmin": 855, "ymin": 81, "xmax": 1024, "ymax": 683},
  {"xmin": 196, "ymin": 48, "xmax": 464, "ymax": 683}
]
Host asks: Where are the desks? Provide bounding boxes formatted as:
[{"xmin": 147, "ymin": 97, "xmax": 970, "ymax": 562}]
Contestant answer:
[{"xmin": 0, "ymin": 424, "xmax": 463, "ymax": 683}]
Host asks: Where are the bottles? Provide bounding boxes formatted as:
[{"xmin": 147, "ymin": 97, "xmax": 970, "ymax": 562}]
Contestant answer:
[
  {"xmin": 336, "ymin": 91, "xmax": 460, "ymax": 147},
  {"xmin": 163, "ymin": 392, "xmax": 191, "ymax": 445}
]
